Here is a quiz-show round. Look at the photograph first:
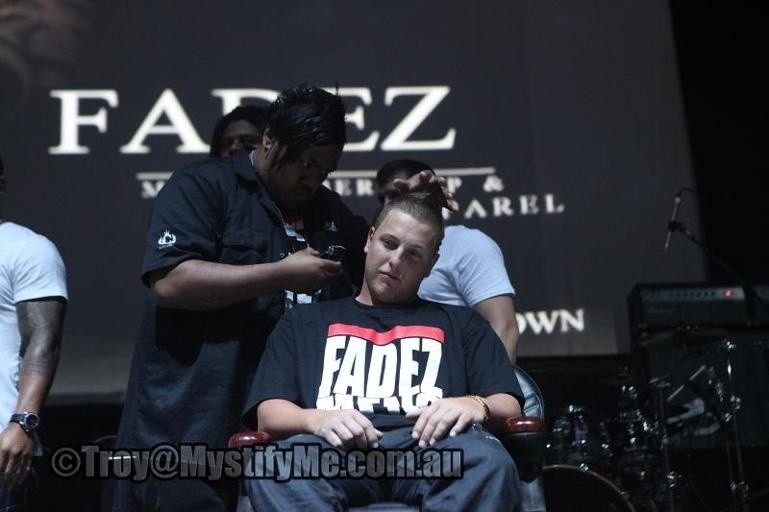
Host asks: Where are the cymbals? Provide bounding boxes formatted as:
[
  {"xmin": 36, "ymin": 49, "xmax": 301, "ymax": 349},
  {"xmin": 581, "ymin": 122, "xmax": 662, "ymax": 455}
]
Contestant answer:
[{"xmin": 639, "ymin": 326, "xmax": 728, "ymax": 348}]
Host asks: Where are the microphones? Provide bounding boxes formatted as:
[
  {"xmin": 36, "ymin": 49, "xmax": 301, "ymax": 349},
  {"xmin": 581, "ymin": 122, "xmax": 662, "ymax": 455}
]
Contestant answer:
[{"xmin": 662, "ymin": 196, "xmax": 682, "ymax": 258}]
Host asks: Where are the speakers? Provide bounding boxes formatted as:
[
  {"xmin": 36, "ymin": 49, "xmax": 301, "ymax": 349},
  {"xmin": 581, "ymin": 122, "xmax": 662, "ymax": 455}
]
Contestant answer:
[{"xmin": 632, "ymin": 329, "xmax": 769, "ymax": 449}]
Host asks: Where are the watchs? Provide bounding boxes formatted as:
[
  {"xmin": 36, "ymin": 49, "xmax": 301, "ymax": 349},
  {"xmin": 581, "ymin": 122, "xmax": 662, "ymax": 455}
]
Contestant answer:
[{"xmin": 10, "ymin": 413, "xmax": 41, "ymax": 433}]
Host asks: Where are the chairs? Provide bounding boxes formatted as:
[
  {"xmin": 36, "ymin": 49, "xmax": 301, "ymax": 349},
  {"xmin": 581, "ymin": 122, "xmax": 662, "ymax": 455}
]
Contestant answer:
[{"xmin": 228, "ymin": 362, "xmax": 545, "ymax": 510}]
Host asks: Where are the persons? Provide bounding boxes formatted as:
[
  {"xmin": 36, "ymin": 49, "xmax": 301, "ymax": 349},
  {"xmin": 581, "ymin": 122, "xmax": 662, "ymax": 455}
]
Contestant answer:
[
  {"xmin": 111, "ymin": 84, "xmax": 461, "ymax": 510},
  {"xmin": 205, "ymin": 105, "xmax": 268, "ymax": 160},
  {"xmin": 373, "ymin": 159, "xmax": 519, "ymax": 372},
  {"xmin": 1, "ymin": 161, "xmax": 71, "ymax": 511},
  {"xmin": 237, "ymin": 196, "xmax": 527, "ymax": 510}
]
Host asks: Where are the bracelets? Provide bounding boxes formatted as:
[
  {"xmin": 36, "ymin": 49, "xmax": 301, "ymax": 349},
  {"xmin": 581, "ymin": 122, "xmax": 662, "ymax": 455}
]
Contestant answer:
[{"xmin": 466, "ymin": 395, "xmax": 490, "ymax": 426}]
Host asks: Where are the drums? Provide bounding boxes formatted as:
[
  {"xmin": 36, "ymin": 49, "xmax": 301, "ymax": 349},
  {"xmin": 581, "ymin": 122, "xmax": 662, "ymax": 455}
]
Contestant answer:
[
  {"xmin": 542, "ymin": 403, "xmax": 616, "ymax": 480},
  {"xmin": 650, "ymin": 364, "xmax": 741, "ymax": 435},
  {"xmin": 599, "ymin": 409, "xmax": 660, "ymax": 479},
  {"xmin": 541, "ymin": 458, "xmax": 658, "ymax": 512}
]
